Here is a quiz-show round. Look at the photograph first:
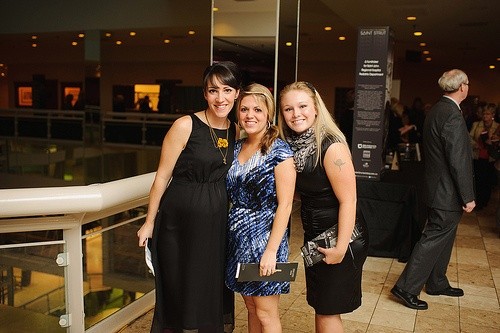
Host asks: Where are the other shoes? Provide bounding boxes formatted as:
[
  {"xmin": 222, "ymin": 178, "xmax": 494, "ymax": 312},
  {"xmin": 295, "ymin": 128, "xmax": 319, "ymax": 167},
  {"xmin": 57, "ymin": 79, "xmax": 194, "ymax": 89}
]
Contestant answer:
[{"xmin": 398, "ymin": 256, "xmax": 408, "ymax": 263}]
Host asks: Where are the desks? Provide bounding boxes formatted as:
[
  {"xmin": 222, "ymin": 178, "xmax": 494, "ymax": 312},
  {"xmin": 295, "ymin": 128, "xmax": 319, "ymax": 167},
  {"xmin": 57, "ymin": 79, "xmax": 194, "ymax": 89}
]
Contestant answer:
[{"xmin": 356, "ymin": 158, "xmax": 429, "ymax": 262}]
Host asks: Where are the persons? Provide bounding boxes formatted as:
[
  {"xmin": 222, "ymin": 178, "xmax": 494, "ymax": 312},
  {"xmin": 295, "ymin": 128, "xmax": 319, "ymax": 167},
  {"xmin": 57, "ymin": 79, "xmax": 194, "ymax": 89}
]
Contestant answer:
[
  {"xmin": 279, "ymin": 80, "xmax": 370, "ymax": 333},
  {"xmin": 386, "ymin": 93, "xmax": 499, "ymax": 208},
  {"xmin": 391, "ymin": 68, "xmax": 477, "ymax": 310},
  {"xmin": 136, "ymin": 60, "xmax": 241, "ymax": 333},
  {"xmin": 227, "ymin": 82, "xmax": 297, "ymax": 333}
]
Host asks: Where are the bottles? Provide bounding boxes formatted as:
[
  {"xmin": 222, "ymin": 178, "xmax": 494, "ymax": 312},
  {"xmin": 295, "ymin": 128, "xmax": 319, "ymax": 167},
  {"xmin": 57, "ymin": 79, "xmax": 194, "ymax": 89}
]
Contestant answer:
[{"xmin": 405, "ymin": 143, "xmax": 412, "ymax": 161}]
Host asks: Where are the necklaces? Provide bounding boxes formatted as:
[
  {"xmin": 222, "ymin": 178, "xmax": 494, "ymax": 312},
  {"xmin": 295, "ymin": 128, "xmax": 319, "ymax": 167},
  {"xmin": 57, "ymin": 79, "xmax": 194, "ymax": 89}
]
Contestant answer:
[{"xmin": 204, "ymin": 105, "xmax": 231, "ymax": 166}]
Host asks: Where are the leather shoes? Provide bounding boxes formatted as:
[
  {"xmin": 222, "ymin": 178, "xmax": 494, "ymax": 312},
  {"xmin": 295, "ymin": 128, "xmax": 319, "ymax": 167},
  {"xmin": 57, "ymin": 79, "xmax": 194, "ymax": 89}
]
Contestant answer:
[
  {"xmin": 391, "ymin": 284, "xmax": 428, "ymax": 310},
  {"xmin": 426, "ymin": 286, "xmax": 464, "ymax": 297}
]
